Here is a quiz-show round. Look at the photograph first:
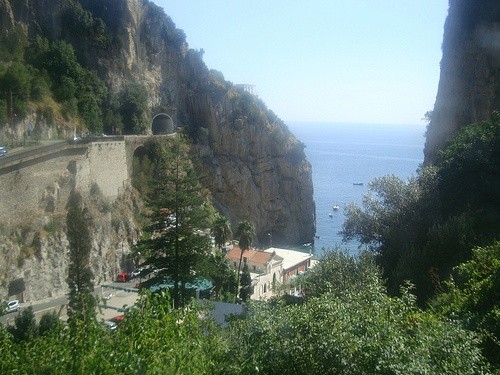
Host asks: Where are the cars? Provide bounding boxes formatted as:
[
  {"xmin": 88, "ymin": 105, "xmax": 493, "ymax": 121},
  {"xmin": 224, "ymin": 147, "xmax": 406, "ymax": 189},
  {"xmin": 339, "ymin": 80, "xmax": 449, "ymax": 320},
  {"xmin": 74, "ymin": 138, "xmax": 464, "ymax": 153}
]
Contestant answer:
[
  {"xmin": 1, "ymin": 300, "xmax": 20, "ymax": 314},
  {"xmin": 131, "ymin": 268, "xmax": 164, "ymax": 289},
  {"xmin": 103, "ymin": 314, "xmax": 125, "ymax": 332},
  {"xmin": 0, "ymin": 145, "xmax": 8, "ymax": 157}
]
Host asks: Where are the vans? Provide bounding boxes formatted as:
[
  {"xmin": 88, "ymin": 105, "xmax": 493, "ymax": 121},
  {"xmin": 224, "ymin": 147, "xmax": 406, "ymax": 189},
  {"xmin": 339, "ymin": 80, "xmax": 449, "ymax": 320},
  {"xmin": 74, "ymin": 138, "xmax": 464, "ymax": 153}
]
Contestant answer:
[{"xmin": 115, "ymin": 274, "xmax": 127, "ymax": 282}]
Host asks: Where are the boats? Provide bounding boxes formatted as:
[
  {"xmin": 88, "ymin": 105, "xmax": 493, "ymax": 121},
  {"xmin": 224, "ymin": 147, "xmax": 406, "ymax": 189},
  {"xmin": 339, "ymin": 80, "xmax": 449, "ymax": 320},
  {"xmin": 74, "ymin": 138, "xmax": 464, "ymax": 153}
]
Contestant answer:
[
  {"xmin": 333, "ymin": 205, "xmax": 339, "ymax": 210},
  {"xmin": 353, "ymin": 182, "xmax": 364, "ymax": 185},
  {"xmin": 328, "ymin": 213, "xmax": 333, "ymax": 217}
]
